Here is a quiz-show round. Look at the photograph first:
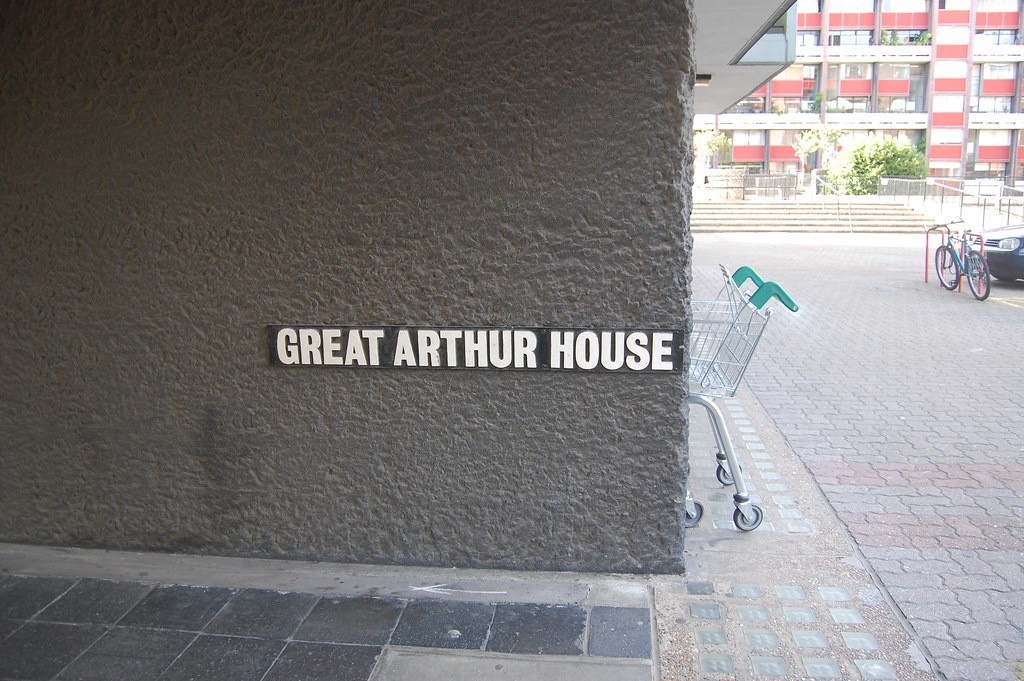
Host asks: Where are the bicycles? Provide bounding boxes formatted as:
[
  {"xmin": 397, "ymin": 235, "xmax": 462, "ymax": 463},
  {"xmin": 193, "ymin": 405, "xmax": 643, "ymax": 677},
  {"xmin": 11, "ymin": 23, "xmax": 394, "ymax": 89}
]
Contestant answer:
[{"xmin": 924, "ymin": 218, "xmax": 993, "ymax": 302}]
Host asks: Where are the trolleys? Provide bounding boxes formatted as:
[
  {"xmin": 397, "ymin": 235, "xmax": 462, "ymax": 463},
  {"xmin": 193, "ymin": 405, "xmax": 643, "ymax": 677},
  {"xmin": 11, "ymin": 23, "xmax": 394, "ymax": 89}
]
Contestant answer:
[{"xmin": 682, "ymin": 266, "xmax": 800, "ymax": 530}]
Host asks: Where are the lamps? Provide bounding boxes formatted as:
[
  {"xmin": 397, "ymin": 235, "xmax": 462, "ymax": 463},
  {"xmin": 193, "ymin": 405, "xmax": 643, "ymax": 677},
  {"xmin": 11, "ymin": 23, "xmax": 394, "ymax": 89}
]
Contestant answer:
[{"xmin": 695, "ymin": 74, "xmax": 711, "ymax": 86}]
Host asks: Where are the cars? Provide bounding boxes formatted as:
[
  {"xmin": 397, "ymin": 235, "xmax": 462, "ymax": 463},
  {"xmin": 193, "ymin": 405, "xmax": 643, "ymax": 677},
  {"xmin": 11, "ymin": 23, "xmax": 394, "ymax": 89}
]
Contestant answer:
[{"xmin": 968, "ymin": 222, "xmax": 1024, "ymax": 283}]
